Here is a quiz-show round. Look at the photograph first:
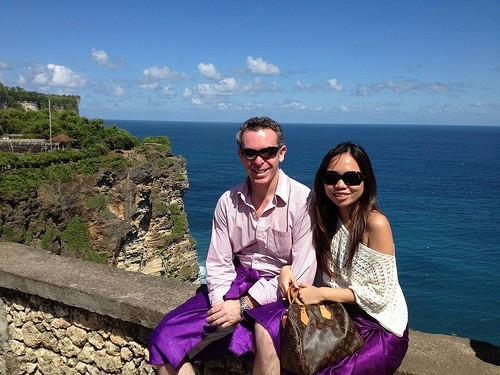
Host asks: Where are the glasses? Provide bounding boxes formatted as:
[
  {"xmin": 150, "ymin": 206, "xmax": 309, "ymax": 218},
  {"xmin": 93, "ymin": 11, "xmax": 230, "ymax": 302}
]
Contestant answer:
[
  {"xmin": 240, "ymin": 146, "xmax": 280, "ymax": 161},
  {"xmin": 323, "ymin": 170, "xmax": 365, "ymax": 186}
]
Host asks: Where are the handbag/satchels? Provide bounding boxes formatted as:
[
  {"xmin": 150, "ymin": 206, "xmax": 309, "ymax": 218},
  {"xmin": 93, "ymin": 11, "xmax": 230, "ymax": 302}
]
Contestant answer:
[{"xmin": 279, "ymin": 282, "xmax": 365, "ymax": 375}]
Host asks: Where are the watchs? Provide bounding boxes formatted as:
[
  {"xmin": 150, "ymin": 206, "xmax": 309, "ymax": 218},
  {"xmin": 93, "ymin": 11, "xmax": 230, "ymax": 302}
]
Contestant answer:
[{"xmin": 238, "ymin": 295, "xmax": 251, "ymax": 318}]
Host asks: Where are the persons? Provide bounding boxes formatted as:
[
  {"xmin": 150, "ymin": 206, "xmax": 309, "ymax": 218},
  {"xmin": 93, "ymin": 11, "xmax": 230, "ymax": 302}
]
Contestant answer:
[
  {"xmin": 276, "ymin": 140, "xmax": 410, "ymax": 375},
  {"xmin": 148, "ymin": 115, "xmax": 318, "ymax": 375}
]
